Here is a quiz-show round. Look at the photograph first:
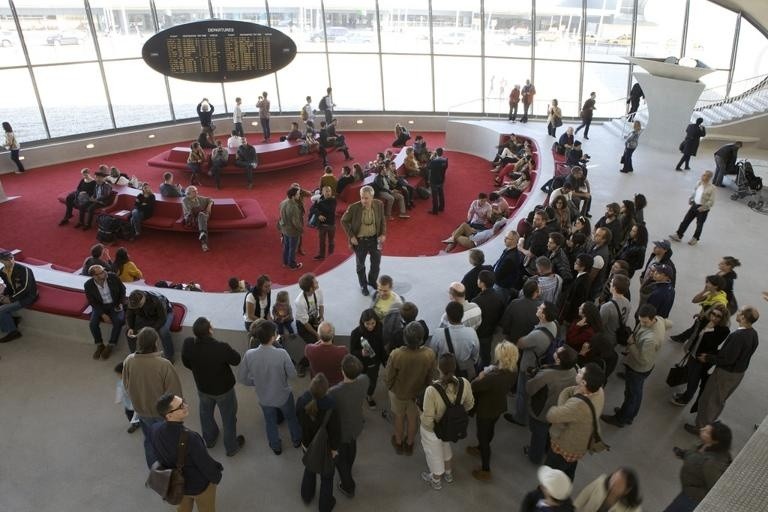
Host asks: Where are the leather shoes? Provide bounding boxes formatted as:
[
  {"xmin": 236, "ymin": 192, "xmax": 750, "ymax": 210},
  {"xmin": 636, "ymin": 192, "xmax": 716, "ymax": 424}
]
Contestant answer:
[
  {"xmin": 0, "ymin": 332, "xmax": 22, "ymax": 343},
  {"xmin": 617, "ymin": 371, "xmax": 625, "ymax": 378},
  {"xmin": 14, "ymin": 315, "xmax": 20, "ymax": 327}
]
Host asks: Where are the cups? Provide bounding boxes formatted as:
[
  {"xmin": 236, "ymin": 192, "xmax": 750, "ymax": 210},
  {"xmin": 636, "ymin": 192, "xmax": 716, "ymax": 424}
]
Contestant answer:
[{"xmin": 377, "ymin": 239, "xmax": 383, "ymax": 251}]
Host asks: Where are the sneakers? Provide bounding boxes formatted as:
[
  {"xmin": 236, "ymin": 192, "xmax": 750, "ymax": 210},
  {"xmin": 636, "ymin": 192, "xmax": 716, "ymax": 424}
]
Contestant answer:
[
  {"xmin": 227, "ymin": 435, "xmax": 245, "ymax": 456},
  {"xmin": 128, "ymin": 425, "xmax": 138, "ymax": 432},
  {"xmin": 688, "ymin": 236, "xmax": 697, "ymax": 245},
  {"xmin": 392, "ymin": 434, "xmax": 414, "ymax": 456},
  {"xmin": 676, "ymin": 164, "xmax": 690, "ymax": 171},
  {"xmin": 443, "ymin": 471, "xmax": 455, "ymax": 483},
  {"xmin": 601, "ymin": 406, "xmax": 633, "ymax": 428},
  {"xmin": 338, "ymin": 479, "xmax": 354, "ymax": 497},
  {"xmin": 686, "ymin": 423, "xmax": 697, "ymax": 434},
  {"xmin": 362, "ymin": 279, "xmax": 378, "ymax": 295},
  {"xmin": 441, "ymin": 237, "xmax": 454, "ymax": 250},
  {"xmin": 387, "ymin": 199, "xmax": 444, "ymax": 221},
  {"xmin": 135, "ymin": 232, "xmax": 140, "ymax": 235},
  {"xmin": 282, "ymin": 243, "xmax": 334, "ymax": 270},
  {"xmin": 199, "ymin": 232, "xmax": 208, "ymax": 252},
  {"xmin": 503, "ymin": 413, "xmax": 527, "ymax": 426},
  {"xmin": 670, "ymin": 391, "xmax": 688, "ymax": 406},
  {"xmin": 366, "ymin": 396, "xmax": 377, "ymax": 409},
  {"xmin": 489, "ymin": 161, "xmax": 502, "ymax": 195},
  {"xmin": 422, "ymin": 472, "xmax": 442, "ymax": 489},
  {"xmin": 59, "ymin": 214, "xmax": 91, "ymax": 231},
  {"xmin": 467, "ymin": 447, "xmax": 481, "ymax": 456},
  {"xmin": 716, "ymin": 184, "xmax": 725, "ymax": 187},
  {"xmin": 620, "ymin": 168, "xmax": 633, "ymax": 172},
  {"xmin": 669, "ymin": 233, "xmax": 681, "ymax": 241},
  {"xmin": 522, "ymin": 445, "xmax": 531, "ymax": 456},
  {"xmin": 473, "ymin": 470, "xmax": 489, "ymax": 480},
  {"xmin": 269, "ymin": 442, "xmax": 281, "ymax": 455},
  {"xmin": 93, "ymin": 343, "xmax": 114, "ymax": 359},
  {"xmin": 328, "ymin": 496, "xmax": 336, "ymax": 512}
]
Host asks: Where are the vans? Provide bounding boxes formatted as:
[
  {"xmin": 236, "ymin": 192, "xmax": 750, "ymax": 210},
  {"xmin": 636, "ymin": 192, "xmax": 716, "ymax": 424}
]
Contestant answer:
[
  {"xmin": 127, "ymin": 15, "xmax": 146, "ymax": 31},
  {"xmin": 310, "ymin": 27, "xmax": 352, "ymax": 43}
]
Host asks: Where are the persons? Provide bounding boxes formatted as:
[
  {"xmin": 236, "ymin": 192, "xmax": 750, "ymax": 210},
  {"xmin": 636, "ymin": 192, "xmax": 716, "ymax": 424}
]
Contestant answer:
[
  {"xmin": 421, "ymin": 117, "xmax": 761, "ymax": 512},
  {"xmin": 0, "ymin": 122, "xmax": 25, "ymax": 174},
  {"xmin": 0, "ymin": 251, "xmax": 40, "ymax": 342},
  {"xmin": 626, "ymin": 83, "xmax": 645, "ymax": 122},
  {"xmin": 255, "ymin": 92, "xmax": 270, "ymax": 143},
  {"xmin": 281, "ymin": 119, "xmax": 448, "ymax": 296},
  {"xmin": 546, "ymin": 98, "xmax": 562, "ymax": 137},
  {"xmin": 233, "ymin": 97, "xmax": 247, "ymax": 137},
  {"xmin": 84, "ymin": 244, "xmax": 244, "ymax": 511},
  {"xmin": 187, "ymin": 129, "xmax": 259, "ymax": 188},
  {"xmin": 574, "ymin": 91, "xmax": 597, "ymax": 140},
  {"xmin": 196, "ymin": 98, "xmax": 217, "ymax": 146},
  {"xmin": 519, "ymin": 79, "xmax": 537, "ymax": 123},
  {"xmin": 303, "ymin": 96, "xmax": 316, "ymax": 132},
  {"xmin": 60, "ymin": 164, "xmax": 215, "ymax": 251},
  {"xmin": 509, "ymin": 84, "xmax": 520, "ymax": 124},
  {"xmin": 235, "ymin": 271, "xmax": 436, "ymax": 511},
  {"xmin": 324, "ymin": 87, "xmax": 337, "ymax": 125}
]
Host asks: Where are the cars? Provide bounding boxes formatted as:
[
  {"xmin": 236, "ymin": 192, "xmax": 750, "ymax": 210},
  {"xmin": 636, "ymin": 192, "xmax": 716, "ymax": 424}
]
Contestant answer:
[
  {"xmin": 501, "ymin": 29, "xmax": 632, "ymax": 46},
  {"xmin": 341, "ymin": 32, "xmax": 375, "ymax": 45},
  {"xmin": 41, "ymin": 20, "xmax": 104, "ymax": 47},
  {"xmin": 429, "ymin": 30, "xmax": 465, "ymax": 45},
  {"xmin": 0, "ymin": 31, "xmax": 16, "ymax": 48}
]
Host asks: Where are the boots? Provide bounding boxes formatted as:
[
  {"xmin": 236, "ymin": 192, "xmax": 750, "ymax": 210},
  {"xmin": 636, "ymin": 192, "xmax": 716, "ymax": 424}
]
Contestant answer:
[{"xmin": 669, "ymin": 329, "xmax": 690, "ymax": 343}]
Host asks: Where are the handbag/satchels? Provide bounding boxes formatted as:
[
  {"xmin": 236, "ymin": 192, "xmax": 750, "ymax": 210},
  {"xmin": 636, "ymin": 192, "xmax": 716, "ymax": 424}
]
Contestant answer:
[
  {"xmin": 726, "ymin": 159, "xmax": 738, "ymax": 174},
  {"xmin": 627, "ymin": 141, "xmax": 636, "ymax": 149},
  {"xmin": 553, "ymin": 117, "xmax": 562, "ymax": 127},
  {"xmin": 680, "ymin": 141, "xmax": 685, "ymax": 150},
  {"xmin": 209, "ymin": 123, "xmax": 216, "ymax": 130},
  {"xmin": 308, "ymin": 214, "xmax": 320, "ymax": 226},
  {"xmin": 666, "ymin": 366, "xmax": 689, "ymax": 386},
  {"xmin": 551, "ymin": 176, "xmax": 566, "ymax": 190},
  {"xmin": 616, "ymin": 326, "xmax": 631, "ymax": 346},
  {"xmin": 417, "ymin": 185, "xmax": 431, "ymax": 199},
  {"xmin": 456, "ymin": 370, "xmax": 471, "ymax": 383},
  {"xmin": 144, "ymin": 461, "xmax": 187, "ymax": 505},
  {"xmin": 727, "ymin": 291, "xmax": 737, "ymax": 315},
  {"xmin": 97, "ymin": 214, "xmax": 133, "ymax": 242},
  {"xmin": 302, "ymin": 427, "xmax": 335, "ymax": 474},
  {"xmin": 589, "ymin": 434, "xmax": 610, "ymax": 455}
]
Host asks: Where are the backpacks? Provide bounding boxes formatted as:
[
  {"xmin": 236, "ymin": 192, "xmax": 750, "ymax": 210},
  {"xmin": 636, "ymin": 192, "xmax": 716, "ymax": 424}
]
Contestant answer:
[
  {"xmin": 319, "ymin": 96, "xmax": 329, "ymax": 110},
  {"xmin": 301, "ymin": 105, "xmax": 310, "ymax": 121},
  {"xmin": 432, "ymin": 377, "xmax": 469, "ymax": 442},
  {"xmin": 533, "ymin": 320, "xmax": 565, "ymax": 363}
]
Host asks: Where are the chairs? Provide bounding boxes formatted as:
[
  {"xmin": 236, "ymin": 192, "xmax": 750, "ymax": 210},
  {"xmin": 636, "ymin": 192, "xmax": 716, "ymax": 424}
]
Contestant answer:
[{"xmin": 438, "ymin": 134, "xmax": 539, "ymax": 255}]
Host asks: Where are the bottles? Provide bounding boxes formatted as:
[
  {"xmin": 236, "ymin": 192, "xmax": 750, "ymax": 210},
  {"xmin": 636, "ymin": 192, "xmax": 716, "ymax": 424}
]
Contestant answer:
[{"xmin": 360, "ymin": 335, "xmax": 376, "ymax": 358}]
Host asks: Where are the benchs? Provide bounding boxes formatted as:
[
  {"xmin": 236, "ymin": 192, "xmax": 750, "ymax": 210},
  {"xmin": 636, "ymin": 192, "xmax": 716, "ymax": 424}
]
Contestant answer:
[
  {"xmin": 312, "ymin": 252, "xmax": 351, "ymax": 277},
  {"xmin": 58, "ymin": 184, "xmax": 267, "ymax": 233},
  {"xmin": 147, "ymin": 134, "xmax": 336, "ymax": 174},
  {"xmin": 335, "ymin": 146, "xmax": 422, "ymax": 219},
  {"xmin": 0, "ymin": 247, "xmax": 187, "ymax": 333}
]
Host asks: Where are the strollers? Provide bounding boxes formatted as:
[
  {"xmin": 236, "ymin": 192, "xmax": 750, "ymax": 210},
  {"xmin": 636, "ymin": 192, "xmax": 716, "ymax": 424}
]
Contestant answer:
[{"xmin": 730, "ymin": 158, "xmax": 765, "ymax": 210}]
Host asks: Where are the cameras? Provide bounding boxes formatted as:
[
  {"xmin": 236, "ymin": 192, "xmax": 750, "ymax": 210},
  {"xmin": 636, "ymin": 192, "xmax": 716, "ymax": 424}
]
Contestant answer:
[
  {"xmin": 312, "ymin": 316, "xmax": 320, "ymax": 324},
  {"xmin": 673, "ymin": 446, "xmax": 686, "ymax": 458},
  {"xmin": 527, "ymin": 366, "xmax": 539, "ymax": 375}
]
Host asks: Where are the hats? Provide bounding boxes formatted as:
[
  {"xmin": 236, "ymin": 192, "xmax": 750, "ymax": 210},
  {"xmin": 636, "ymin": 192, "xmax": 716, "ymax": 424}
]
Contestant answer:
[
  {"xmin": 538, "ymin": 465, "xmax": 574, "ymax": 501},
  {"xmin": 652, "ymin": 240, "xmax": 670, "ymax": 250},
  {"xmin": 0, "ymin": 251, "xmax": 13, "ymax": 260},
  {"xmin": 654, "ymin": 264, "xmax": 673, "ymax": 278},
  {"xmin": 128, "ymin": 290, "xmax": 144, "ymax": 309}
]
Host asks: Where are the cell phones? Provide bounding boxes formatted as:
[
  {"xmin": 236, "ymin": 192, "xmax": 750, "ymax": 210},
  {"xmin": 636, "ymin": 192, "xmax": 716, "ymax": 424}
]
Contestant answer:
[
  {"xmin": 116, "ymin": 306, "xmax": 120, "ymax": 310},
  {"xmin": 130, "ymin": 334, "xmax": 137, "ymax": 338}
]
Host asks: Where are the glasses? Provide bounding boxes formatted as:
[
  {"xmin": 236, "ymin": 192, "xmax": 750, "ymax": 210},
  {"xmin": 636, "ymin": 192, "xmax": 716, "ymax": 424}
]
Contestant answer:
[
  {"xmin": 711, "ymin": 311, "xmax": 722, "ymax": 318},
  {"xmin": 363, "ymin": 213, "xmax": 373, "ymax": 225},
  {"xmin": 169, "ymin": 399, "xmax": 186, "ymax": 413}
]
